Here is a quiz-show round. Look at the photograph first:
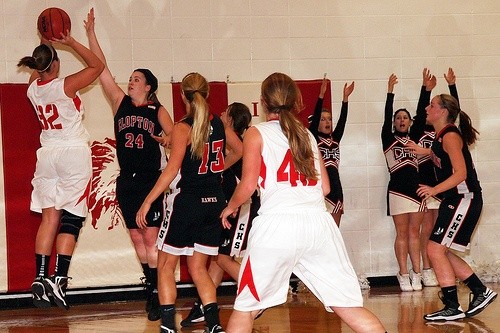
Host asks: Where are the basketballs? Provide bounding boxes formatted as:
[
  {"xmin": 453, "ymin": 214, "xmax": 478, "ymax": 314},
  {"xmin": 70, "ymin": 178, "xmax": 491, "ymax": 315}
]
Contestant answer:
[{"xmin": 38, "ymin": 8, "xmax": 71, "ymax": 41}]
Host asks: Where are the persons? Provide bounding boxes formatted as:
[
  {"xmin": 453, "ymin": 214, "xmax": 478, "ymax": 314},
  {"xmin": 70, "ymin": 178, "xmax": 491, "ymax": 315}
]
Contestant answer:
[
  {"xmin": 17, "ymin": 29, "xmax": 106, "ymax": 310},
  {"xmin": 135, "ymin": 68, "xmax": 497, "ymax": 332},
  {"xmin": 83, "ymin": 7, "xmax": 175, "ymax": 322}
]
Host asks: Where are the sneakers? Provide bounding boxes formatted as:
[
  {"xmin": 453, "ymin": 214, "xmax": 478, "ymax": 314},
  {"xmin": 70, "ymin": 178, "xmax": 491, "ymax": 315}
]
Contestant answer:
[
  {"xmin": 44, "ymin": 275, "xmax": 73, "ymax": 310},
  {"xmin": 148, "ymin": 292, "xmax": 161, "ymax": 321},
  {"xmin": 421, "ymin": 268, "xmax": 436, "ymax": 287},
  {"xmin": 397, "ymin": 272, "xmax": 413, "ymax": 291},
  {"xmin": 180, "ymin": 300, "xmax": 220, "ymax": 327},
  {"xmin": 431, "ymin": 268, "xmax": 439, "ymax": 285},
  {"xmin": 409, "ymin": 269, "xmax": 423, "ymax": 290},
  {"xmin": 203, "ymin": 324, "xmax": 226, "ymax": 333},
  {"xmin": 31, "ymin": 277, "xmax": 51, "ymax": 308},
  {"xmin": 423, "ymin": 291, "xmax": 466, "ymax": 320},
  {"xmin": 160, "ymin": 324, "xmax": 178, "ymax": 333},
  {"xmin": 465, "ymin": 285, "xmax": 498, "ymax": 318},
  {"xmin": 254, "ymin": 308, "xmax": 266, "ymax": 320},
  {"xmin": 140, "ymin": 277, "xmax": 149, "ymax": 312}
]
